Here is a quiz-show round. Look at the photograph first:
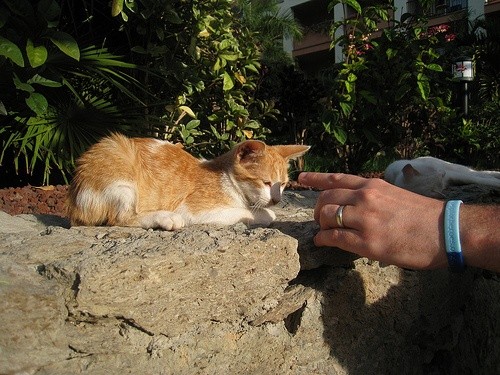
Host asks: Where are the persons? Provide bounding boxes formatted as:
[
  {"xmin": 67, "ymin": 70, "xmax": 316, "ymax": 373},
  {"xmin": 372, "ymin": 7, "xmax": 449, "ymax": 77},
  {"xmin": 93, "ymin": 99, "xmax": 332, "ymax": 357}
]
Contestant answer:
[{"xmin": 295, "ymin": 168, "xmax": 499, "ymax": 281}]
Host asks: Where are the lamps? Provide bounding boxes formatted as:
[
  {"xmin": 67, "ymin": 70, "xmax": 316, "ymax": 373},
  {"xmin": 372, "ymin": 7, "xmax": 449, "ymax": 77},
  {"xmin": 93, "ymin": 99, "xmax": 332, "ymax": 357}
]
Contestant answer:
[{"xmin": 449, "ymin": 51, "xmax": 477, "ymax": 120}]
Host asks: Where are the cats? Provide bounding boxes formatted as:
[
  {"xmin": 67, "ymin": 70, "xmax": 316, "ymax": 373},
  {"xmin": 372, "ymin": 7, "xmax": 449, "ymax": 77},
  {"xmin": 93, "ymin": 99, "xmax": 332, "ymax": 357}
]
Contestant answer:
[
  {"xmin": 383, "ymin": 156, "xmax": 500, "ymax": 199},
  {"xmin": 66, "ymin": 132, "xmax": 312, "ymax": 227}
]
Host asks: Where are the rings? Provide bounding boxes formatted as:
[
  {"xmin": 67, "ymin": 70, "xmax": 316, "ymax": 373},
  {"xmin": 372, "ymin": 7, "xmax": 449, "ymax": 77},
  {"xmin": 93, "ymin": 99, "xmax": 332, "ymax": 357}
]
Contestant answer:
[{"xmin": 334, "ymin": 204, "xmax": 347, "ymax": 229}]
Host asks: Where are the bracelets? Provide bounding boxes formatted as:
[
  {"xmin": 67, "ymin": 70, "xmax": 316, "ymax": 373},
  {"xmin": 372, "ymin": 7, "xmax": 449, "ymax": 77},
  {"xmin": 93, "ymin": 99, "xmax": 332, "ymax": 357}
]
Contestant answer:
[{"xmin": 442, "ymin": 198, "xmax": 475, "ymax": 277}]
